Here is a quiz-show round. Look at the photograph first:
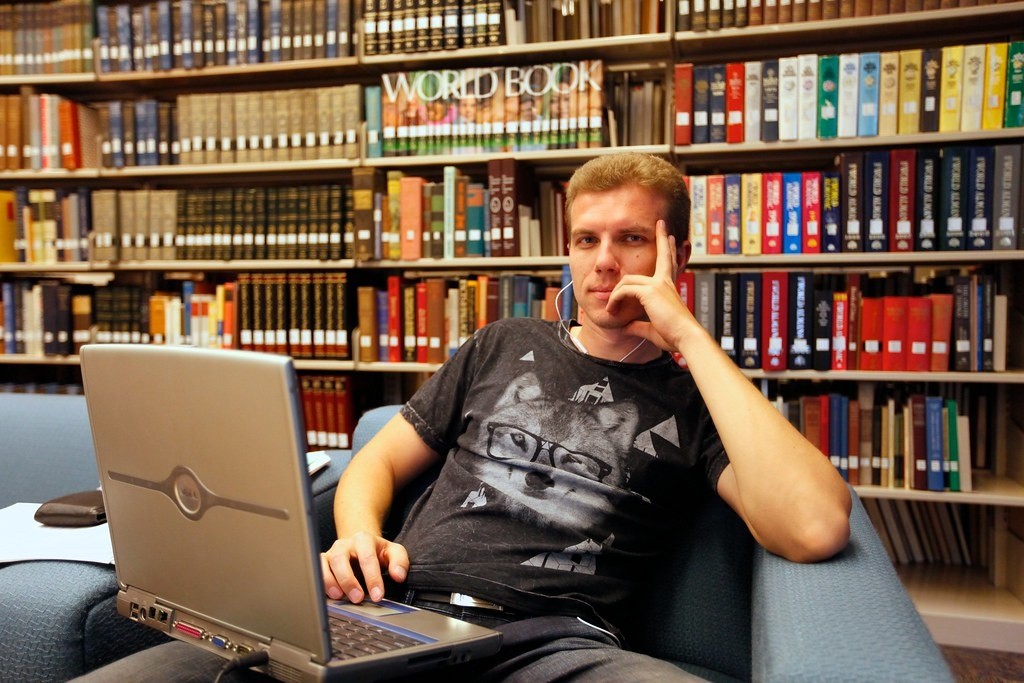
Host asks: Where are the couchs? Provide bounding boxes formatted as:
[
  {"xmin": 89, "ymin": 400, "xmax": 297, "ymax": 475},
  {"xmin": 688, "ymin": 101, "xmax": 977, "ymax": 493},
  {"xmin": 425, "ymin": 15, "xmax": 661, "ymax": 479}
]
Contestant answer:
[
  {"xmin": 300, "ymin": 403, "xmax": 953, "ymax": 683},
  {"xmin": 0, "ymin": 392, "xmax": 176, "ymax": 683}
]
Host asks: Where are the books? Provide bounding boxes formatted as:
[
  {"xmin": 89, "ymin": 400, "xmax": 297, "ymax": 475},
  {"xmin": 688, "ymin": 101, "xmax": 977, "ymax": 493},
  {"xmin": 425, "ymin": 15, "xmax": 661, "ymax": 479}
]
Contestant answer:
[
  {"xmin": 0, "ymin": 265, "xmax": 1013, "ymax": 570},
  {"xmin": 0, "ymin": 0, "xmax": 1024, "ymax": 262}
]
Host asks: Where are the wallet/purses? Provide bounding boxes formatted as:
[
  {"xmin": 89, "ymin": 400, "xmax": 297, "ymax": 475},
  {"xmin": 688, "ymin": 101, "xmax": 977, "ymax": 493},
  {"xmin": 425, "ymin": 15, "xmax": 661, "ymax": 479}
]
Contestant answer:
[{"xmin": 34, "ymin": 491, "xmax": 108, "ymax": 526}]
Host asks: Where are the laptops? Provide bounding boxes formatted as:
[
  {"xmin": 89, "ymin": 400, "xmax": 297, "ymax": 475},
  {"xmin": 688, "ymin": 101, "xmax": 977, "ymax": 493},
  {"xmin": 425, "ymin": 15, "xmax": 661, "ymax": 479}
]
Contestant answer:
[{"xmin": 79, "ymin": 344, "xmax": 503, "ymax": 683}]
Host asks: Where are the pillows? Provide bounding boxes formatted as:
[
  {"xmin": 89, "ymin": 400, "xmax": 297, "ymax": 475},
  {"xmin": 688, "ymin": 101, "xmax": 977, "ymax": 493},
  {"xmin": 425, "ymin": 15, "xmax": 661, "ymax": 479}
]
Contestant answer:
[{"xmin": 645, "ymin": 496, "xmax": 750, "ymax": 681}]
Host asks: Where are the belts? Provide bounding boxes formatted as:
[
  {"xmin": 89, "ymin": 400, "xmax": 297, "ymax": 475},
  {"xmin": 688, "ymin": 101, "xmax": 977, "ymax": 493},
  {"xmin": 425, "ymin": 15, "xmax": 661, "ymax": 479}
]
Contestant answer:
[{"xmin": 413, "ymin": 589, "xmax": 514, "ymax": 612}]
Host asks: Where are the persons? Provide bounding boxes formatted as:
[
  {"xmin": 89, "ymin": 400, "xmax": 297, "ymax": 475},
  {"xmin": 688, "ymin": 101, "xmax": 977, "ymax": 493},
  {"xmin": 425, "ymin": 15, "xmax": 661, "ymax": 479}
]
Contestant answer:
[{"xmin": 66, "ymin": 154, "xmax": 855, "ymax": 682}]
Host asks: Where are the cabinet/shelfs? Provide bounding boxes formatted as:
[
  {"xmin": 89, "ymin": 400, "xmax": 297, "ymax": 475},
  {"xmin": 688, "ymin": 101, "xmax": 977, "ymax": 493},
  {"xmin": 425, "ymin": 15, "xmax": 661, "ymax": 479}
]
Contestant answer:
[{"xmin": 1, "ymin": 1, "xmax": 1024, "ymax": 653}]
